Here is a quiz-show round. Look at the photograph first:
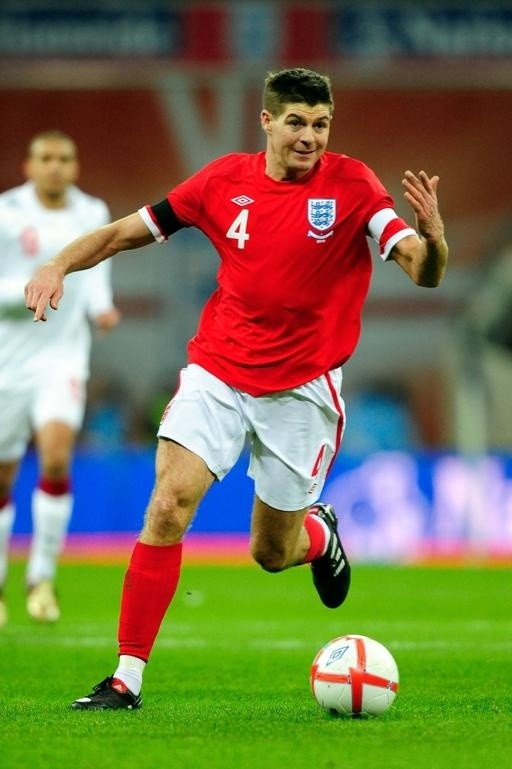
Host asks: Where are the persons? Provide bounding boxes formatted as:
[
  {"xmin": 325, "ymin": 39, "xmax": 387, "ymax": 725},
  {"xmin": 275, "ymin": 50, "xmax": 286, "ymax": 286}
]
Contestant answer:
[
  {"xmin": 22, "ymin": 65, "xmax": 447, "ymax": 711},
  {"xmin": 0, "ymin": 128, "xmax": 121, "ymax": 631}
]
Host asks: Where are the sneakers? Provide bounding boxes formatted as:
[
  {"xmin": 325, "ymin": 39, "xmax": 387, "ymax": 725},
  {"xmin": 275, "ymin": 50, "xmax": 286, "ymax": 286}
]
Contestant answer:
[
  {"xmin": 307, "ymin": 503, "xmax": 352, "ymax": 608},
  {"xmin": 25, "ymin": 580, "xmax": 61, "ymax": 622},
  {"xmin": 69, "ymin": 673, "xmax": 146, "ymax": 715}
]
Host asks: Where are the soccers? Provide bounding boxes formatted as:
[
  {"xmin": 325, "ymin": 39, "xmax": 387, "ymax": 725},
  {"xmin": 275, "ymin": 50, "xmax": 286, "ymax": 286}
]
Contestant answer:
[{"xmin": 310, "ymin": 635, "xmax": 399, "ymax": 717}]
